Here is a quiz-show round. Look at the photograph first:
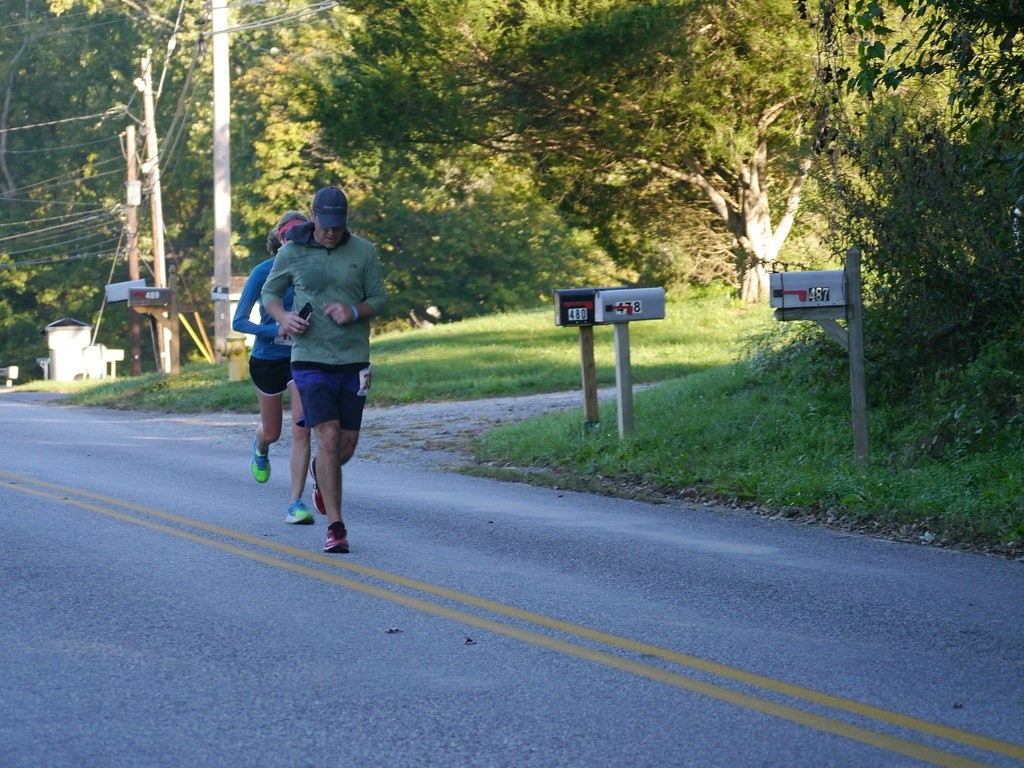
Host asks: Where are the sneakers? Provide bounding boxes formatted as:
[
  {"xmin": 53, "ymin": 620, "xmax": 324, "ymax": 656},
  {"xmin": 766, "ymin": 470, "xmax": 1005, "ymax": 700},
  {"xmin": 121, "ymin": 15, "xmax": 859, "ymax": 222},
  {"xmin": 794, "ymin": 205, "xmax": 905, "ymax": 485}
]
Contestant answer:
[
  {"xmin": 285, "ymin": 499, "xmax": 315, "ymax": 524},
  {"xmin": 308, "ymin": 457, "xmax": 327, "ymax": 516},
  {"xmin": 323, "ymin": 528, "xmax": 349, "ymax": 553},
  {"xmin": 249, "ymin": 434, "xmax": 271, "ymax": 485}
]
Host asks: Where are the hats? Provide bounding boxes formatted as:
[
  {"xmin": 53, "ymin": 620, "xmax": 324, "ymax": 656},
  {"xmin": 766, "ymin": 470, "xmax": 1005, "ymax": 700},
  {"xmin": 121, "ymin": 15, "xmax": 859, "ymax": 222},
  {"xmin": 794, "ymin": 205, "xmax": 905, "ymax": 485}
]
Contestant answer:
[{"xmin": 313, "ymin": 186, "xmax": 348, "ymax": 228}]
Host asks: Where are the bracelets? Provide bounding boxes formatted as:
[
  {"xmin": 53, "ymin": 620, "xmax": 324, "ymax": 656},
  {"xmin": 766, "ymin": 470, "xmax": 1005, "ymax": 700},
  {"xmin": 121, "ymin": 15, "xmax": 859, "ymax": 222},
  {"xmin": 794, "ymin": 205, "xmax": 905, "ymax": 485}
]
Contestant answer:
[{"xmin": 350, "ymin": 304, "xmax": 359, "ymax": 321}]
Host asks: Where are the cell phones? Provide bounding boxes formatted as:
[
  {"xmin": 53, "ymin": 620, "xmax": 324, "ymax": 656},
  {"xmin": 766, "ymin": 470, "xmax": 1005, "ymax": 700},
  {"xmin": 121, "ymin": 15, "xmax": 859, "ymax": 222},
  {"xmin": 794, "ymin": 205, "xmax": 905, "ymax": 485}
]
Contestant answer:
[{"xmin": 298, "ymin": 302, "xmax": 313, "ymax": 325}]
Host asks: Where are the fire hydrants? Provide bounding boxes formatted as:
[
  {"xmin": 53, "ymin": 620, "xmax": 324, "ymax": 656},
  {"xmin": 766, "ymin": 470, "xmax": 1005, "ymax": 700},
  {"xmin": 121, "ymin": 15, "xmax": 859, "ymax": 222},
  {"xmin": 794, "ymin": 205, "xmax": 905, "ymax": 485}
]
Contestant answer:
[{"xmin": 217, "ymin": 330, "xmax": 253, "ymax": 384}]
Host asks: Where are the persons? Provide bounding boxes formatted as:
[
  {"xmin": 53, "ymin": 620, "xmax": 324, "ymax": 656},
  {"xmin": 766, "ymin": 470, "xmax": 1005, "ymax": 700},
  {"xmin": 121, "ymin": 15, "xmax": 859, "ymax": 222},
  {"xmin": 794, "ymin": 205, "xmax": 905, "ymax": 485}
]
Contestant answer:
[
  {"xmin": 232, "ymin": 212, "xmax": 315, "ymax": 524},
  {"xmin": 261, "ymin": 186, "xmax": 387, "ymax": 552}
]
